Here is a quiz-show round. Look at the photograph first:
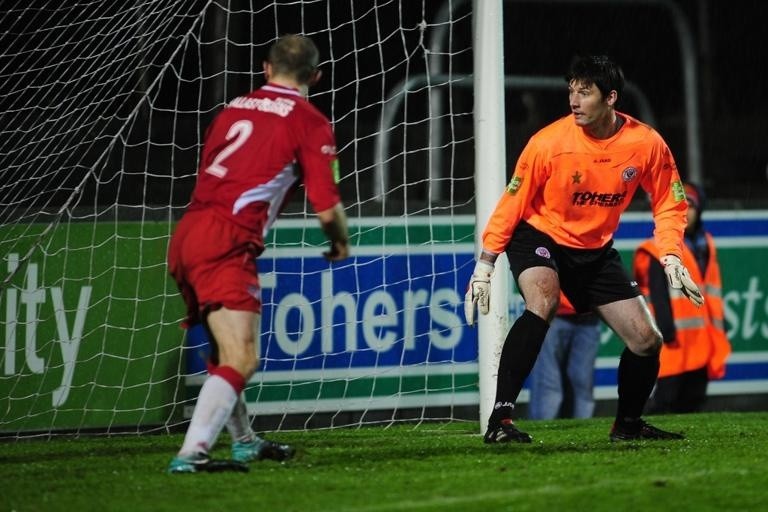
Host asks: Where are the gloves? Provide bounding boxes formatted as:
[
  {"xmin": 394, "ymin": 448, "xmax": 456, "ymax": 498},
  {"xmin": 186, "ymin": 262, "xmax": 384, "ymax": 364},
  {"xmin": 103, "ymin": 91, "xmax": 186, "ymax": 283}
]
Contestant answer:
[
  {"xmin": 463, "ymin": 258, "xmax": 497, "ymax": 326},
  {"xmin": 660, "ymin": 254, "xmax": 705, "ymax": 308}
]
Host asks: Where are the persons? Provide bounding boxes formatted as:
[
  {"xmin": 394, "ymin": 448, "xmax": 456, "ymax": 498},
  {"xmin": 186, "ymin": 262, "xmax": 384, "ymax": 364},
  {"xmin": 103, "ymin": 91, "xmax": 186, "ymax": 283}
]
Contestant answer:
[
  {"xmin": 164, "ymin": 33, "xmax": 355, "ymax": 474},
  {"xmin": 629, "ymin": 175, "xmax": 733, "ymax": 416},
  {"xmin": 524, "ymin": 286, "xmax": 605, "ymax": 425},
  {"xmin": 460, "ymin": 49, "xmax": 706, "ymax": 447}
]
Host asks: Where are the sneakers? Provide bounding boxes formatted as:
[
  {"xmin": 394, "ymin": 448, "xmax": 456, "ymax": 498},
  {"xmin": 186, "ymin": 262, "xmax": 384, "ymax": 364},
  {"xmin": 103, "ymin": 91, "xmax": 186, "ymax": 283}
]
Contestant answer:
[
  {"xmin": 609, "ymin": 417, "xmax": 687, "ymax": 442},
  {"xmin": 482, "ymin": 416, "xmax": 533, "ymax": 445},
  {"xmin": 167, "ymin": 453, "xmax": 249, "ymax": 474},
  {"xmin": 231, "ymin": 434, "xmax": 296, "ymax": 463}
]
